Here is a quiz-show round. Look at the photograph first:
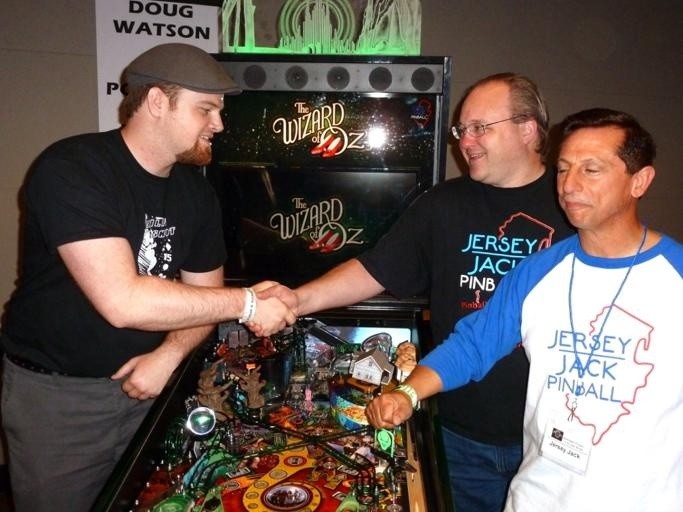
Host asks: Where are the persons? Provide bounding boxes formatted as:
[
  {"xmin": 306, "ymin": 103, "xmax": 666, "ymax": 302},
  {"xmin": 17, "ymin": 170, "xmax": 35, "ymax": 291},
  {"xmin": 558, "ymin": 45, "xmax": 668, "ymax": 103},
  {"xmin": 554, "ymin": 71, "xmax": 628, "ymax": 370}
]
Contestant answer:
[
  {"xmin": 365, "ymin": 109, "xmax": 681, "ymax": 512},
  {"xmin": 0, "ymin": 43, "xmax": 299, "ymax": 512},
  {"xmin": 246, "ymin": 69, "xmax": 577, "ymax": 512}
]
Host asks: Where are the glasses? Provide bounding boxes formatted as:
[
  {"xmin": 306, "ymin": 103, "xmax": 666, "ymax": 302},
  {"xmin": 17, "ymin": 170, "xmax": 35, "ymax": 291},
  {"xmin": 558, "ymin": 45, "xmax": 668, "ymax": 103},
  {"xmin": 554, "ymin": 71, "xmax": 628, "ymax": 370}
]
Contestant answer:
[{"xmin": 451, "ymin": 114, "xmax": 528, "ymax": 140}]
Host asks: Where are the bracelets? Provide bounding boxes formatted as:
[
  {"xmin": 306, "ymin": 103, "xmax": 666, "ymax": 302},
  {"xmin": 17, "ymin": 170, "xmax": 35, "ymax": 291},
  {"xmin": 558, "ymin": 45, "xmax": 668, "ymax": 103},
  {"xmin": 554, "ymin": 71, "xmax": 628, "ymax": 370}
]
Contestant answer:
[
  {"xmin": 392, "ymin": 385, "xmax": 421, "ymax": 411},
  {"xmin": 237, "ymin": 285, "xmax": 257, "ymax": 328}
]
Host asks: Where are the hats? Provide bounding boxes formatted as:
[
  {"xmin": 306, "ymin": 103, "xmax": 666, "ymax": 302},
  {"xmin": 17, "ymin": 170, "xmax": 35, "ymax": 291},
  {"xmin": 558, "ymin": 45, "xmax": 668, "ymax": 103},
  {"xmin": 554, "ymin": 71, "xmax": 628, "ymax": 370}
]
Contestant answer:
[{"xmin": 120, "ymin": 42, "xmax": 244, "ymax": 95}]
